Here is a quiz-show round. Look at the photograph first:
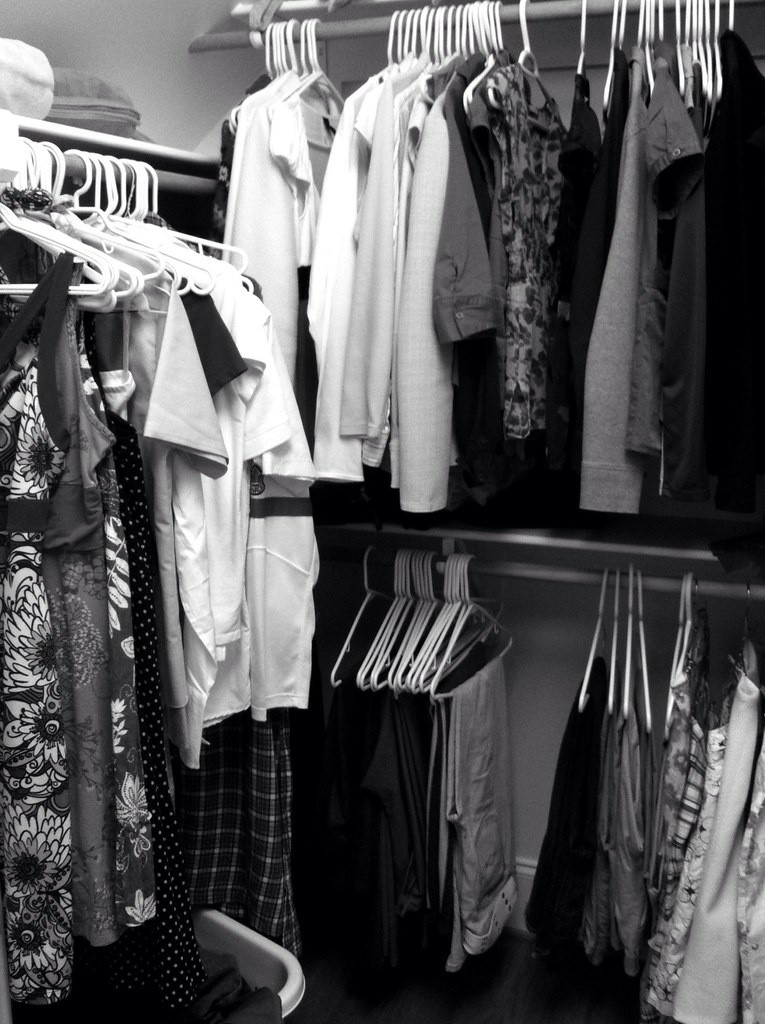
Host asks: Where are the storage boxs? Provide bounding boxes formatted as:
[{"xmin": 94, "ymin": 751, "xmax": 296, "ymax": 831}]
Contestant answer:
[{"xmin": 194, "ymin": 909, "xmax": 307, "ymax": 1024}]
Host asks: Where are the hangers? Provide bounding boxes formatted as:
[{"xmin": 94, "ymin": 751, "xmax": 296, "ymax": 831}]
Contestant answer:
[
  {"xmin": 0, "ymin": 136, "xmax": 260, "ymax": 311},
  {"xmin": 329, "ymin": 545, "xmax": 755, "ymax": 735},
  {"xmin": 264, "ymin": 0, "xmax": 737, "ymax": 114}
]
